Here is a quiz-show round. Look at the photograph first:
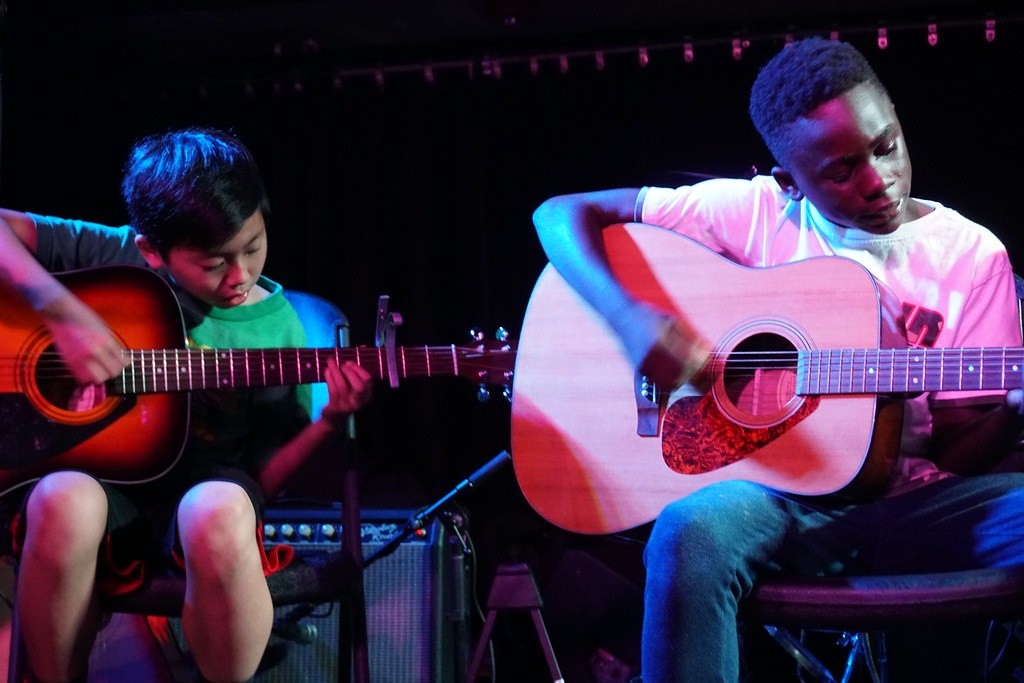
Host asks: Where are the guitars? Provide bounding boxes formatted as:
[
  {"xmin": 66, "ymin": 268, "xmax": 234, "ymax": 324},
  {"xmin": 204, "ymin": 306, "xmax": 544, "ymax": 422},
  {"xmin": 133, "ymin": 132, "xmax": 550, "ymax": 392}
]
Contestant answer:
[
  {"xmin": 0, "ymin": 261, "xmax": 519, "ymax": 504},
  {"xmin": 507, "ymin": 221, "xmax": 1023, "ymax": 540}
]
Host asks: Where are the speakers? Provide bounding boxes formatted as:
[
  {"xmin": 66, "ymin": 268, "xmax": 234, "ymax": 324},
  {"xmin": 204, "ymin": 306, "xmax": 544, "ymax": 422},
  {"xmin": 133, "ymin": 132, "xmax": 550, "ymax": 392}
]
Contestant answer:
[{"xmin": 166, "ymin": 508, "xmax": 472, "ymax": 683}]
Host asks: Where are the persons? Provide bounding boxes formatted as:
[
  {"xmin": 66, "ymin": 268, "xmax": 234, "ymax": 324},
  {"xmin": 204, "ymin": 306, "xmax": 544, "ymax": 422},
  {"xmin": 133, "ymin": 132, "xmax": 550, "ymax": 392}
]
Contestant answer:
[
  {"xmin": 0, "ymin": 127, "xmax": 371, "ymax": 683},
  {"xmin": 533, "ymin": 38, "xmax": 1024, "ymax": 683}
]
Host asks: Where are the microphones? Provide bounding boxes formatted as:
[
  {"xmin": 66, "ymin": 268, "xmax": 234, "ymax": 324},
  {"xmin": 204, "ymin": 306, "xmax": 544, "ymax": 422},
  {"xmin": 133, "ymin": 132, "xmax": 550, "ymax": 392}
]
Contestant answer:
[{"xmin": 271, "ymin": 617, "xmax": 317, "ymax": 646}]
